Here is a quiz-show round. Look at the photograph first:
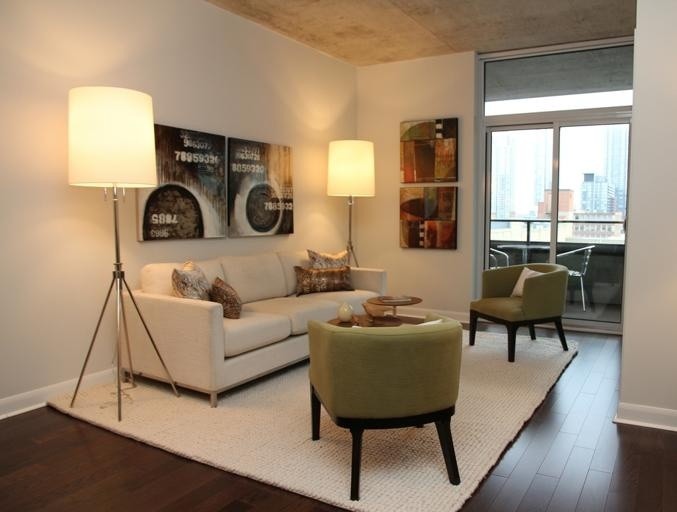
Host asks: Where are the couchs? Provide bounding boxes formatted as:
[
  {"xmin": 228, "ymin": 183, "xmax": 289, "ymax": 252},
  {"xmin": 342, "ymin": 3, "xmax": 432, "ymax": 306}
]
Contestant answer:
[{"xmin": 114, "ymin": 247, "xmax": 388, "ymax": 409}]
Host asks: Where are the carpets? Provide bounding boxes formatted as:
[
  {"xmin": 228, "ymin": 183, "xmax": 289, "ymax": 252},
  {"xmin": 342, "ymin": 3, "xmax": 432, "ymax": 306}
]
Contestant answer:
[{"xmin": 44, "ymin": 329, "xmax": 579, "ymax": 512}]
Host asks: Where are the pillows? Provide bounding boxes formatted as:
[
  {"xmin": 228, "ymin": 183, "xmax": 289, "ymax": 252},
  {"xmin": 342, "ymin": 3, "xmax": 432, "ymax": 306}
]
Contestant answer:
[
  {"xmin": 351, "ymin": 318, "xmax": 442, "ymax": 330},
  {"xmin": 510, "ymin": 267, "xmax": 547, "ymax": 298},
  {"xmin": 205, "ymin": 277, "xmax": 241, "ymax": 320},
  {"xmin": 307, "ymin": 249, "xmax": 351, "ymax": 273},
  {"xmin": 170, "ymin": 260, "xmax": 208, "ymax": 300},
  {"xmin": 292, "ymin": 266, "xmax": 355, "ymax": 297}
]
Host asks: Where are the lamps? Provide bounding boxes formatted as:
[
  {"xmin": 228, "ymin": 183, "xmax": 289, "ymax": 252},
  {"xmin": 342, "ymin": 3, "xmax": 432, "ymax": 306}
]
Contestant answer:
[
  {"xmin": 327, "ymin": 139, "xmax": 375, "ymax": 267},
  {"xmin": 66, "ymin": 85, "xmax": 182, "ymax": 422}
]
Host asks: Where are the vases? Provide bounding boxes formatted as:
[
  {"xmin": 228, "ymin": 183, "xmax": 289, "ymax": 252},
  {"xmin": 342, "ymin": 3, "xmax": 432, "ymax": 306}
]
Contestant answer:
[{"xmin": 337, "ymin": 301, "xmax": 355, "ymax": 323}]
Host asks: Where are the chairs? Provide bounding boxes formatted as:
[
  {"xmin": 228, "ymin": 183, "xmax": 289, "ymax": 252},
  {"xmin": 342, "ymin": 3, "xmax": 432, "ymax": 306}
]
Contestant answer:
[
  {"xmin": 468, "ymin": 262, "xmax": 569, "ymax": 361},
  {"xmin": 305, "ymin": 315, "xmax": 470, "ymax": 498},
  {"xmin": 548, "ymin": 245, "xmax": 595, "ymax": 311},
  {"xmin": 488, "ymin": 247, "xmax": 508, "ymax": 269}
]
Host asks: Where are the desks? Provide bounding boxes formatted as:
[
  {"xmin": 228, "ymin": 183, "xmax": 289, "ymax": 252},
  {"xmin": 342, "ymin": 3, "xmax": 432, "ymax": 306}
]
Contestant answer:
[{"xmin": 496, "ymin": 242, "xmax": 550, "ymax": 264}]
